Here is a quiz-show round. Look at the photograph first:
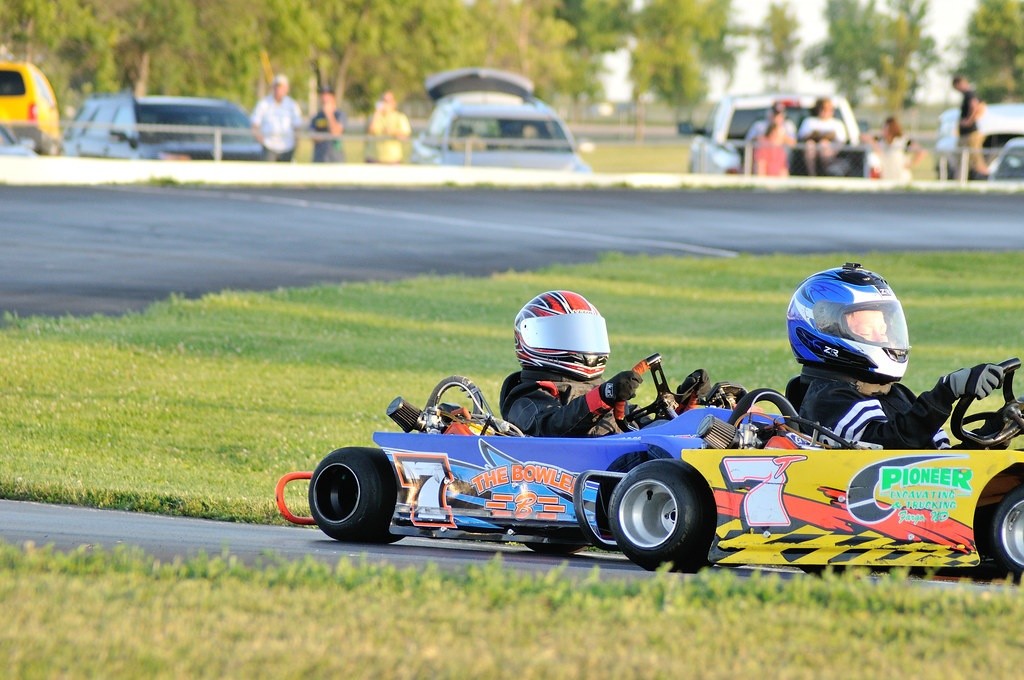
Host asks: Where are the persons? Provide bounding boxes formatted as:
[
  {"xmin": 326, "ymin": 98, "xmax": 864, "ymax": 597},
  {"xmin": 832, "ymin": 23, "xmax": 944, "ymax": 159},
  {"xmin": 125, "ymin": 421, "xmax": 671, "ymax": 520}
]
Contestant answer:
[
  {"xmin": 307, "ymin": 87, "xmax": 352, "ymax": 163},
  {"xmin": 368, "ymin": 89, "xmax": 410, "ymax": 164},
  {"xmin": 950, "ymin": 73, "xmax": 991, "ymax": 181},
  {"xmin": 859, "ymin": 116, "xmax": 926, "ymax": 181},
  {"xmin": 499, "ymin": 289, "xmax": 708, "ymax": 439},
  {"xmin": 786, "ymin": 260, "xmax": 1006, "ymax": 450},
  {"xmin": 248, "ymin": 72, "xmax": 303, "ymax": 161},
  {"xmin": 747, "ymin": 97, "xmax": 847, "ymax": 176}
]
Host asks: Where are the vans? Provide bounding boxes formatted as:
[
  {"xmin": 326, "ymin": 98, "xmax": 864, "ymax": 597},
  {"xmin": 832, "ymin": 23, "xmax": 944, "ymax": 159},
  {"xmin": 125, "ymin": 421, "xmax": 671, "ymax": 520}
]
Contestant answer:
[{"xmin": 1, "ymin": 58, "xmax": 64, "ymax": 154}]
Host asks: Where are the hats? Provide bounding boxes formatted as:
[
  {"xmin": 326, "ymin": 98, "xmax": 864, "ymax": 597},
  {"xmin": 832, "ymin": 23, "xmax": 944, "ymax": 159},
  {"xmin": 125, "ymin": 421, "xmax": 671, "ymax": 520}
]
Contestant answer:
[
  {"xmin": 773, "ymin": 101, "xmax": 785, "ymax": 114},
  {"xmin": 273, "ymin": 74, "xmax": 288, "ymax": 85},
  {"xmin": 318, "ymin": 84, "xmax": 335, "ymax": 95}
]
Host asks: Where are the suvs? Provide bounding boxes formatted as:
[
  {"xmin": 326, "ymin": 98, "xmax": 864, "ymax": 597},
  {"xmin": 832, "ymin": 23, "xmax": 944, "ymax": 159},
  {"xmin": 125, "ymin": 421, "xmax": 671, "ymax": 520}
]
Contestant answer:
[{"xmin": 62, "ymin": 92, "xmax": 268, "ymax": 162}]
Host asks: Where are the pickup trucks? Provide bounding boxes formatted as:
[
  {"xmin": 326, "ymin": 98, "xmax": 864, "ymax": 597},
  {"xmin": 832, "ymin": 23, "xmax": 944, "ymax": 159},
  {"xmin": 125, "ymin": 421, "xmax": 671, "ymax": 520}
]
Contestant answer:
[{"xmin": 676, "ymin": 87, "xmax": 885, "ymax": 179}]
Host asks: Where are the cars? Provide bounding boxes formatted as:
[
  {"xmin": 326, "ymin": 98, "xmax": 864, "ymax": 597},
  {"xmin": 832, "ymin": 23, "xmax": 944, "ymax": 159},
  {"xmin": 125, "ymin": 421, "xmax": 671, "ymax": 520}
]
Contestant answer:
[
  {"xmin": 406, "ymin": 66, "xmax": 590, "ymax": 175},
  {"xmin": 933, "ymin": 100, "xmax": 1024, "ymax": 181}
]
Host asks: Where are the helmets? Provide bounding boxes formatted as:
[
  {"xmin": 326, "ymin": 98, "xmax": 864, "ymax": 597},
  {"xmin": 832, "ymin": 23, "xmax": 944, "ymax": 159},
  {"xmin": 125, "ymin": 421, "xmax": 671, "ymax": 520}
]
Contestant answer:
[
  {"xmin": 787, "ymin": 262, "xmax": 912, "ymax": 382},
  {"xmin": 514, "ymin": 290, "xmax": 611, "ymax": 379}
]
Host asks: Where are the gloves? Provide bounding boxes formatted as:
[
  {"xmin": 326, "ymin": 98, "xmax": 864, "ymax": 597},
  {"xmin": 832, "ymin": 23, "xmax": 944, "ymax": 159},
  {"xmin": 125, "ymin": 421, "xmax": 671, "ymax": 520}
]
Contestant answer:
[
  {"xmin": 677, "ymin": 368, "xmax": 711, "ymax": 415},
  {"xmin": 941, "ymin": 363, "xmax": 1005, "ymax": 400},
  {"xmin": 586, "ymin": 370, "xmax": 643, "ymax": 417}
]
